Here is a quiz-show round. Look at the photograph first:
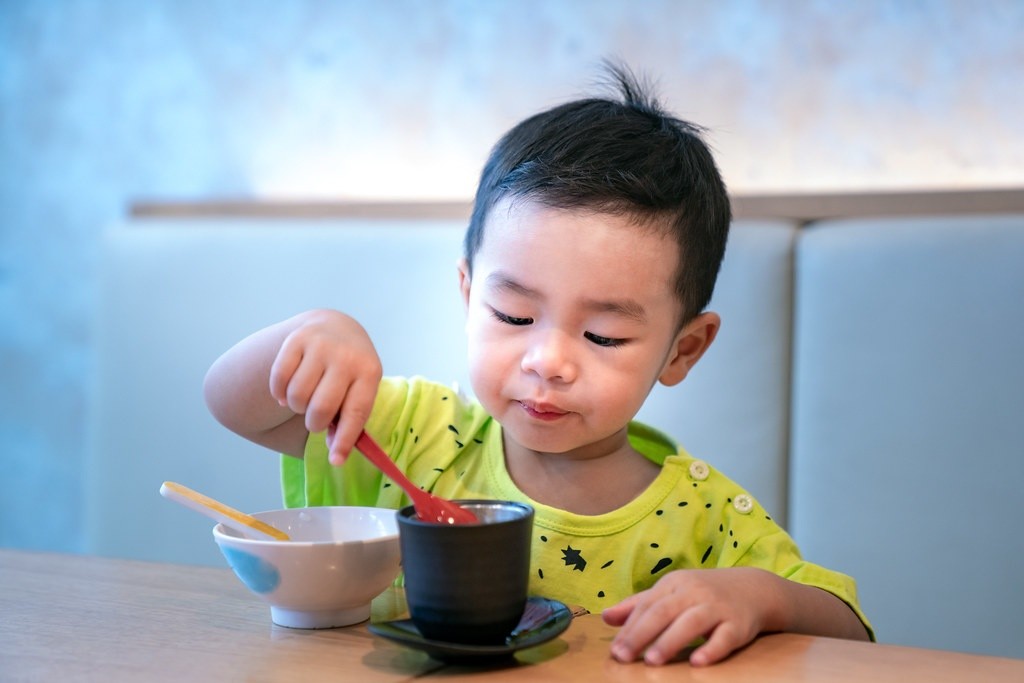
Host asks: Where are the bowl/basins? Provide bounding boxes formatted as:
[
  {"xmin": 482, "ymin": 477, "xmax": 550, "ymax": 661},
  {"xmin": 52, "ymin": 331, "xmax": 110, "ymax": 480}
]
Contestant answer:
[{"xmin": 213, "ymin": 505, "xmax": 404, "ymax": 629}]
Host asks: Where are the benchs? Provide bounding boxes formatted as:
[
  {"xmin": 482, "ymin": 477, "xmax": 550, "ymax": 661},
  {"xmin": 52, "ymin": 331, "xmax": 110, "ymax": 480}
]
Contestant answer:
[{"xmin": 79, "ymin": 184, "xmax": 1024, "ymax": 660}]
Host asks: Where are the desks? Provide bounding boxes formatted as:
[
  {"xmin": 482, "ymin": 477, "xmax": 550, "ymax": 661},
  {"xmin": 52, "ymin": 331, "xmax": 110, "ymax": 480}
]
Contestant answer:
[{"xmin": 0, "ymin": 544, "xmax": 1024, "ymax": 683}]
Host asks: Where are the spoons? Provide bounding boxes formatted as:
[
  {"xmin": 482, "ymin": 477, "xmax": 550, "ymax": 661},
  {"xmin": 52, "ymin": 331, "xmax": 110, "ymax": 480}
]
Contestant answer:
[{"xmin": 330, "ymin": 414, "xmax": 478, "ymax": 526}]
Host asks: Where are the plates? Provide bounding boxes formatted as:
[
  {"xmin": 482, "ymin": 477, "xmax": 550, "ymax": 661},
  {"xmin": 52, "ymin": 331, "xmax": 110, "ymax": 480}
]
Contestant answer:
[{"xmin": 370, "ymin": 595, "xmax": 573, "ymax": 668}]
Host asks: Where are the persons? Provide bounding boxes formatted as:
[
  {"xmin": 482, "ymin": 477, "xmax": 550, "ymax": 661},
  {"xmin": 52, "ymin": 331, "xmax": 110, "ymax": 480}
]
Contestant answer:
[{"xmin": 202, "ymin": 54, "xmax": 879, "ymax": 667}]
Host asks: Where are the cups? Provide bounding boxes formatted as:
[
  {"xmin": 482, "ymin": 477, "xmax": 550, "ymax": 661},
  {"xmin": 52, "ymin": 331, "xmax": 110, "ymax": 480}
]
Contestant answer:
[{"xmin": 395, "ymin": 498, "xmax": 535, "ymax": 643}]
